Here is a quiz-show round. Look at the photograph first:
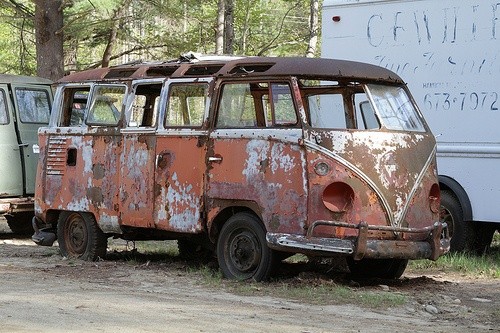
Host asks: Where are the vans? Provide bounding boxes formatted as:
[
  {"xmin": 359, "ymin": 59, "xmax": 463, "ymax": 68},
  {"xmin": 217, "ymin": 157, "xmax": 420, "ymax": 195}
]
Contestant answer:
[
  {"xmin": 0, "ymin": 74, "xmax": 57, "ymax": 234},
  {"xmin": 30, "ymin": 56, "xmax": 450, "ymax": 284}
]
